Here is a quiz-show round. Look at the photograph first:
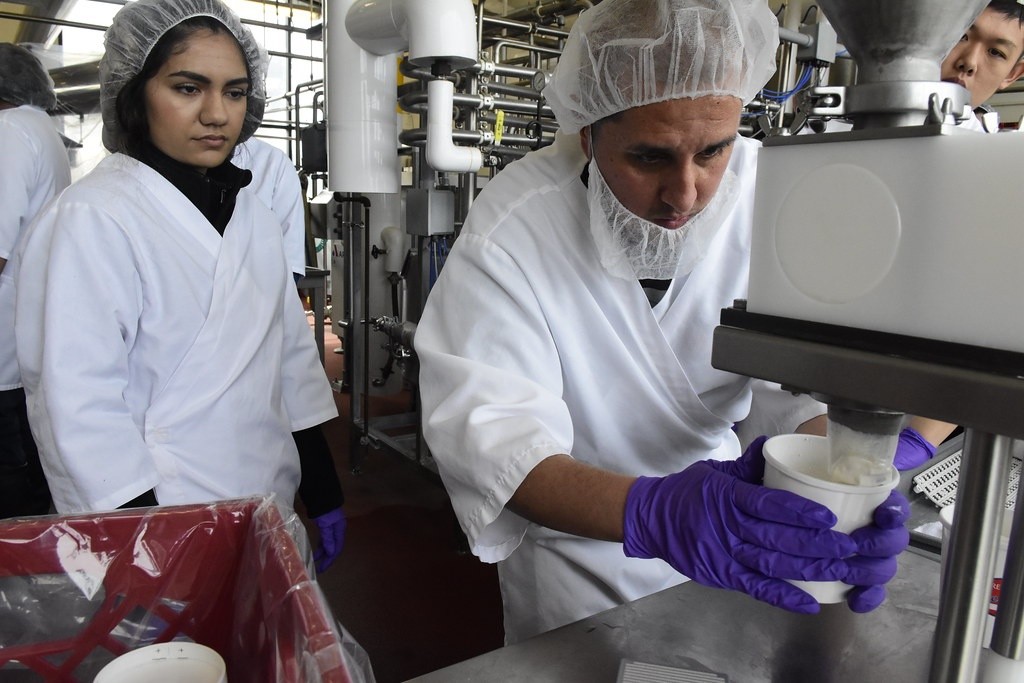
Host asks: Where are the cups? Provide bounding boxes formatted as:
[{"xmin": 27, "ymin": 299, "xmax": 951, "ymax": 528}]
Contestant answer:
[
  {"xmin": 91, "ymin": 642, "xmax": 226, "ymax": 683},
  {"xmin": 939, "ymin": 505, "xmax": 1024, "ymax": 661},
  {"xmin": 762, "ymin": 432, "xmax": 901, "ymax": 602}
]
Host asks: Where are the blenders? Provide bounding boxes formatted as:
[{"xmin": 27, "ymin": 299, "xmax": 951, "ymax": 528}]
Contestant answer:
[{"xmin": 711, "ymin": 1, "xmax": 1024, "ymax": 440}]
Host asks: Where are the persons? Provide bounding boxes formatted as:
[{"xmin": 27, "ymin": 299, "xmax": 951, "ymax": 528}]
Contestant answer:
[
  {"xmin": 940, "ymin": 1, "xmax": 1024, "ymax": 111},
  {"xmin": 412, "ymin": 0, "xmax": 915, "ymax": 647},
  {"xmin": 231, "ymin": 137, "xmax": 307, "ymax": 283},
  {"xmin": 17, "ymin": 1, "xmax": 350, "ymax": 572},
  {"xmin": 0, "ymin": 42, "xmax": 73, "ymax": 518},
  {"xmin": 893, "ymin": 412, "xmax": 958, "ymax": 471}
]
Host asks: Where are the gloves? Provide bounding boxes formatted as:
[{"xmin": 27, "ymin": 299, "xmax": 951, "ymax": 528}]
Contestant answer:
[
  {"xmin": 308, "ymin": 509, "xmax": 346, "ymax": 572},
  {"xmin": 891, "ymin": 424, "xmax": 937, "ymax": 471},
  {"xmin": 845, "ymin": 490, "xmax": 912, "ymax": 615},
  {"xmin": 621, "ymin": 434, "xmax": 858, "ymax": 617}
]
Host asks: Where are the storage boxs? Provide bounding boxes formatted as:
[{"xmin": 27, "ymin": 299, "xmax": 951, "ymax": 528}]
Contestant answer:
[{"xmin": 0, "ymin": 496, "xmax": 345, "ymax": 683}]
